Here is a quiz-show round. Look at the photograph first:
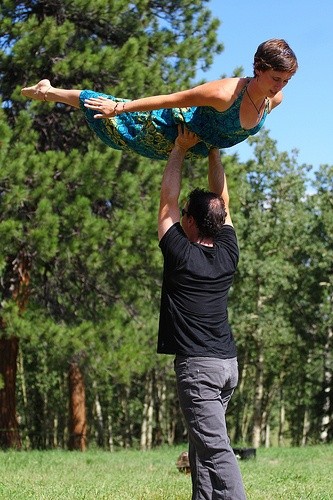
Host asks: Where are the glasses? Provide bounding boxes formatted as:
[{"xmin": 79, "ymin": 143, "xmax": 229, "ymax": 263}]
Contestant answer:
[{"xmin": 181, "ymin": 209, "xmax": 187, "ymax": 217}]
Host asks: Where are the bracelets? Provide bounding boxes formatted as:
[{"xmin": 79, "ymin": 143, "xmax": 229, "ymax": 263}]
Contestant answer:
[
  {"xmin": 114, "ymin": 102, "xmax": 121, "ymax": 116},
  {"xmin": 122, "ymin": 102, "xmax": 127, "ymax": 112}
]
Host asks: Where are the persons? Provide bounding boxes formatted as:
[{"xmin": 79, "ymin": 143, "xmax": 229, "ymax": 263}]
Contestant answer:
[
  {"xmin": 156, "ymin": 124, "xmax": 248, "ymax": 500},
  {"xmin": 20, "ymin": 38, "xmax": 298, "ymax": 161}
]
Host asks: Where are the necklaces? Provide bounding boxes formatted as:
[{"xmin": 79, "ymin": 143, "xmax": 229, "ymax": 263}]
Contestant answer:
[{"xmin": 245, "ymin": 87, "xmax": 268, "ymax": 119}]
